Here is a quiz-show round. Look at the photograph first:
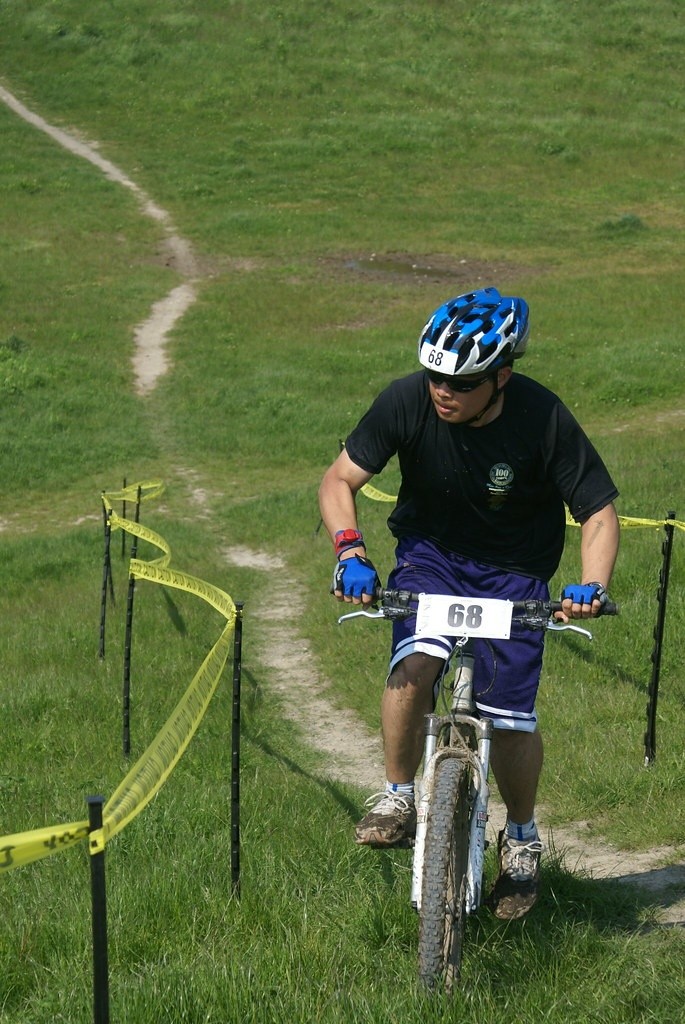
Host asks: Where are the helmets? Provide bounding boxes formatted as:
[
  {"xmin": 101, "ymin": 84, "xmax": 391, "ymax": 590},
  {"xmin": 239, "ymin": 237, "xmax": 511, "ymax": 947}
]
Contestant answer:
[{"xmin": 417, "ymin": 287, "xmax": 531, "ymax": 375}]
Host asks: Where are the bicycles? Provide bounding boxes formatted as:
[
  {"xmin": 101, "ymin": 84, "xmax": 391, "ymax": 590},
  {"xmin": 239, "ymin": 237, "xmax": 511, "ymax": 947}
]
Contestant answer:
[{"xmin": 326, "ymin": 582, "xmax": 624, "ymax": 1001}]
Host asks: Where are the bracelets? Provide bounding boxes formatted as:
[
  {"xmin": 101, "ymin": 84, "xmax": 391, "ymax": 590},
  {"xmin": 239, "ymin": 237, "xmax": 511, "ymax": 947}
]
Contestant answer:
[{"xmin": 334, "ymin": 530, "xmax": 367, "ymax": 558}]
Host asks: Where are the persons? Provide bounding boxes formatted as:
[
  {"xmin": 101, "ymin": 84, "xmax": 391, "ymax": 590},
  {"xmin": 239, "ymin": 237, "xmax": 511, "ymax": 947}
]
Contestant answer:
[{"xmin": 318, "ymin": 287, "xmax": 623, "ymax": 921}]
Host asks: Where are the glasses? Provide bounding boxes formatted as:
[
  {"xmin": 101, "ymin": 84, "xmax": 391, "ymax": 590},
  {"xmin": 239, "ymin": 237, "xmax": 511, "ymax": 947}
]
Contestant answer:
[{"xmin": 421, "ymin": 363, "xmax": 500, "ymax": 391}]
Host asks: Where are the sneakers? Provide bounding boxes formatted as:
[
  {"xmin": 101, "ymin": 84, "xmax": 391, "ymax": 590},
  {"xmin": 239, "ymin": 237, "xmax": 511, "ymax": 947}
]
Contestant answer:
[
  {"xmin": 492, "ymin": 833, "xmax": 546, "ymax": 918},
  {"xmin": 353, "ymin": 791, "xmax": 415, "ymax": 847}
]
